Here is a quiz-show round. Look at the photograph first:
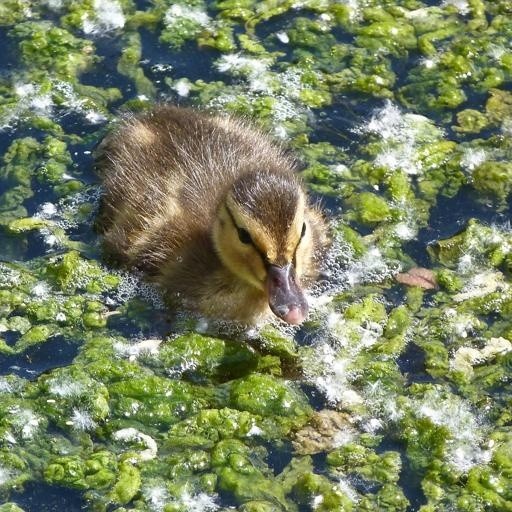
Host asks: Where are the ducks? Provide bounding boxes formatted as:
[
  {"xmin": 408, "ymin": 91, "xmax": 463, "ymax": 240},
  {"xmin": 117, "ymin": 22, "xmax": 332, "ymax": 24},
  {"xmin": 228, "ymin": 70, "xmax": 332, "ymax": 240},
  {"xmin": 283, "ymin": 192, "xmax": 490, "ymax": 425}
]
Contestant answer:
[{"xmin": 91, "ymin": 100, "xmax": 332, "ymax": 325}]
